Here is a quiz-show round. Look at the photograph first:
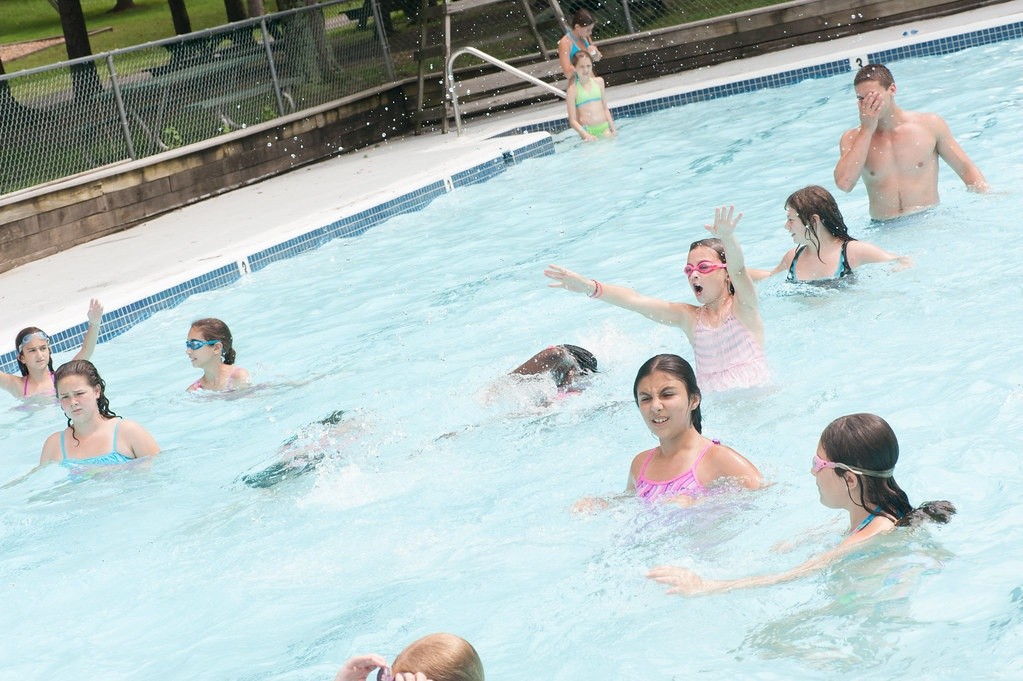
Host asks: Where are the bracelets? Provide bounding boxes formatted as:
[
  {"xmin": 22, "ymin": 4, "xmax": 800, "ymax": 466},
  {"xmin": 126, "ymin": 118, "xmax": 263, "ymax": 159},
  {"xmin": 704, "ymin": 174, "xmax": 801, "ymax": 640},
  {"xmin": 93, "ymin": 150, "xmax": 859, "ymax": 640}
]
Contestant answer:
[{"xmin": 587, "ymin": 280, "xmax": 603, "ymax": 300}]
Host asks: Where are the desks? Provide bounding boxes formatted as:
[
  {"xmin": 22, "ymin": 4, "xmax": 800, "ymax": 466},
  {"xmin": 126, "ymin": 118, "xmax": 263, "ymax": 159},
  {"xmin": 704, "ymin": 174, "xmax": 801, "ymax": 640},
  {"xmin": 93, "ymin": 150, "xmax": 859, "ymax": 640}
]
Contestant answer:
[{"xmin": 99, "ymin": 50, "xmax": 285, "ymax": 153}]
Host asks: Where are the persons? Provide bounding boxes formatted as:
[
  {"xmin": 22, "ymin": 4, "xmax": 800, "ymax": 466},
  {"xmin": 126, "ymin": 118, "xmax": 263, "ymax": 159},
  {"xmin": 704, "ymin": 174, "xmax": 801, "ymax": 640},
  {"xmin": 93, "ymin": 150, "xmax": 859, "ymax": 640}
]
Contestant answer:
[
  {"xmin": 627, "ymin": 353, "xmax": 762, "ymax": 502},
  {"xmin": 567, "ymin": 50, "xmax": 616, "ymax": 142},
  {"xmin": 810, "ymin": 413, "xmax": 915, "ymax": 551},
  {"xmin": 747, "ymin": 184, "xmax": 903, "ymax": 285},
  {"xmin": 834, "ymin": 64, "xmax": 988, "ymax": 223},
  {"xmin": 0, "ymin": 298, "xmax": 104, "ymax": 402},
  {"xmin": 334, "ymin": 632, "xmax": 486, "ymax": 681},
  {"xmin": 543, "ymin": 204, "xmax": 776, "ymax": 395},
  {"xmin": 558, "ymin": 10, "xmax": 602, "ymax": 87},
  {"xmin": 40, "ymin": 360, "xmax": 161, "ymax": 469},
  {"xmin": 509, "ymin": 344, "xmax": 597, "ymax": 401},
  {"xmin": 186, "ymin": 318, "xmax": 249, "ymax": 392}
]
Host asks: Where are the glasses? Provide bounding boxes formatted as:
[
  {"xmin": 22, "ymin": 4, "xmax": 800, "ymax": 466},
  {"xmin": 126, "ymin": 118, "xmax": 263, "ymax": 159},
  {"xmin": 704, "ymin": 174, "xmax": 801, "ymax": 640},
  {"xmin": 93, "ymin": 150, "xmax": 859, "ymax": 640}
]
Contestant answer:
[
  {"xmin": 684, "ymin": 262, "xmax": 726, "ymax": 276},
  {"xmin": 19, "ymin": 332, "xmax": 50, "ymax": 353},
  {"xmin": 812, "ymin": 457, "xmax": 834, "ymax": 474},
  {"xmin": 186, "ymin": 340, "xmax": 220, "ymax": 350}
]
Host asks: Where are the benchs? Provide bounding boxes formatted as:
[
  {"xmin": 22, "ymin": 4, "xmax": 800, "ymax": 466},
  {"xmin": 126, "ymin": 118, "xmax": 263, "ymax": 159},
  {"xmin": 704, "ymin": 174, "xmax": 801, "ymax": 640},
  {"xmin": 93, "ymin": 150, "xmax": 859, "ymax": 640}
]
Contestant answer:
[{"xmin": 187, "ymin": 76, "xmax": 300, "ymax": 136}]
